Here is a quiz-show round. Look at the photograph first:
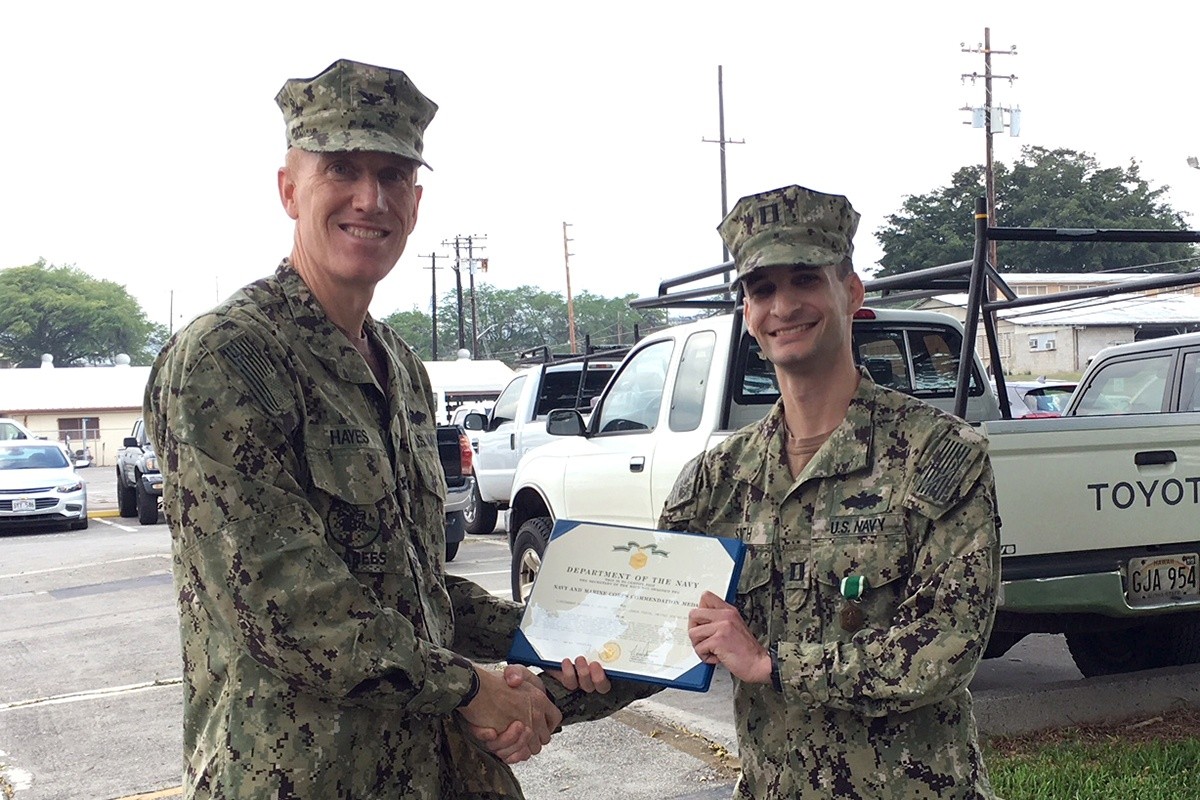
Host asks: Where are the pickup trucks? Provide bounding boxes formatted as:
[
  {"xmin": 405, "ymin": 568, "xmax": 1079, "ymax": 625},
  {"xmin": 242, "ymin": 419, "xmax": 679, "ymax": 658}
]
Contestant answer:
[
  {"xmin": 115, "ymin": 416, "xmax": 164, "ymax": 525},
  {"xmin": 464, "ymin": 361, "xmax": 623, "ymax": 535},
  {"xmin": 434, "ymin": 424, "xmax": 474, "ymax": 562},
  {"xmin": 508, "ymin": 304, "xmax": 1200, "ymax": 679}
]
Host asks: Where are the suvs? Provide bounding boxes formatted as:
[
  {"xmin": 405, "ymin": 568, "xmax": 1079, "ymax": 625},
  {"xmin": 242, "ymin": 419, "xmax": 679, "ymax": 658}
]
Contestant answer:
[
  {"xmin": 1059, "ymin": 330, "xmax": 1200, "ymax": 417},
  {"xmin": 0, "ymin": 417, "xmax": 71, "ymax": 458}
]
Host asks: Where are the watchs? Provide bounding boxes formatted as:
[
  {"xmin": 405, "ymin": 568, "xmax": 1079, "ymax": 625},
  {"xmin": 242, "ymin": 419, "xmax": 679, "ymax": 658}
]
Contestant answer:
[
  {"xmin": 457, "ymin": 670, "xmax": 480, "ymax": 707},
  {"xmin": 767, "ymin": 644, "xmax": 783, "ymax": 693}
]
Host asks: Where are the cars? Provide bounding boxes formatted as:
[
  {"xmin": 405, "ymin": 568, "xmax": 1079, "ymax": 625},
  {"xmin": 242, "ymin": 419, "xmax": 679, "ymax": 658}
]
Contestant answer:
[
  {"xmin": 989, "ymin": 376, "xmax": 1120, "ymax": 419},
  {"xmin": 0, "ymin": 439, "xmax": 90, "ymax": 531}
]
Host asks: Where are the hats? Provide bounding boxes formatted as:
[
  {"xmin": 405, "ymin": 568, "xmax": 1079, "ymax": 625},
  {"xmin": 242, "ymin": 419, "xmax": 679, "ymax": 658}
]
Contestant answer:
[
  {"xmin": 274, "ymin": 58, "xmax": 440, "ymax": 173},
  {"xmin": 718, "ymin": 185, "xmax": 861, "ymax": 292}
]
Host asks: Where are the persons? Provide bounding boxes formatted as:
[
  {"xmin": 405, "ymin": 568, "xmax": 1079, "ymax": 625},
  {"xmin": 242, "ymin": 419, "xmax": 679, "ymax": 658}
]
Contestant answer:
[
  {"xmin": 470, "ymin": 184, "xmax": 1002, "ymax": 800},
  {"xmin": 143, "ymin": 59, "xmax": 611, "ymax": 800}
]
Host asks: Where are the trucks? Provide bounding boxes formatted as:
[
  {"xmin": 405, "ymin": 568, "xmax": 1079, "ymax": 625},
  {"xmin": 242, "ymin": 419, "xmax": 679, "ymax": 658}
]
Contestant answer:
[{"xmin": 448, "ymin": 399, "xmax": 495, "ymax": 453}]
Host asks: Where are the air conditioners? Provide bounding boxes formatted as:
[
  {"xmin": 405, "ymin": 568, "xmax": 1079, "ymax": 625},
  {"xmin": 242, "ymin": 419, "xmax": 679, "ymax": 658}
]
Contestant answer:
[
  {"xmin": 1046, "ymin": 342, "xmax": 1053, "ymax": 349},
  {"xmin": 1029, "ymin": 341, "xmax": 1037, "ymax": 346}
]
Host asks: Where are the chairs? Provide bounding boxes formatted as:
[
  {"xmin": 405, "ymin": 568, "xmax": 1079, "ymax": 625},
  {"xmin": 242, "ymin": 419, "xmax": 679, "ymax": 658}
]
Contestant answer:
[
  {"xmin": 29, "ymin": 453, "xmax": 49, "ymax": 466},
  {"xmin": 866, "ymin": 359, "xmax": 893, "ymax": 385}
]
